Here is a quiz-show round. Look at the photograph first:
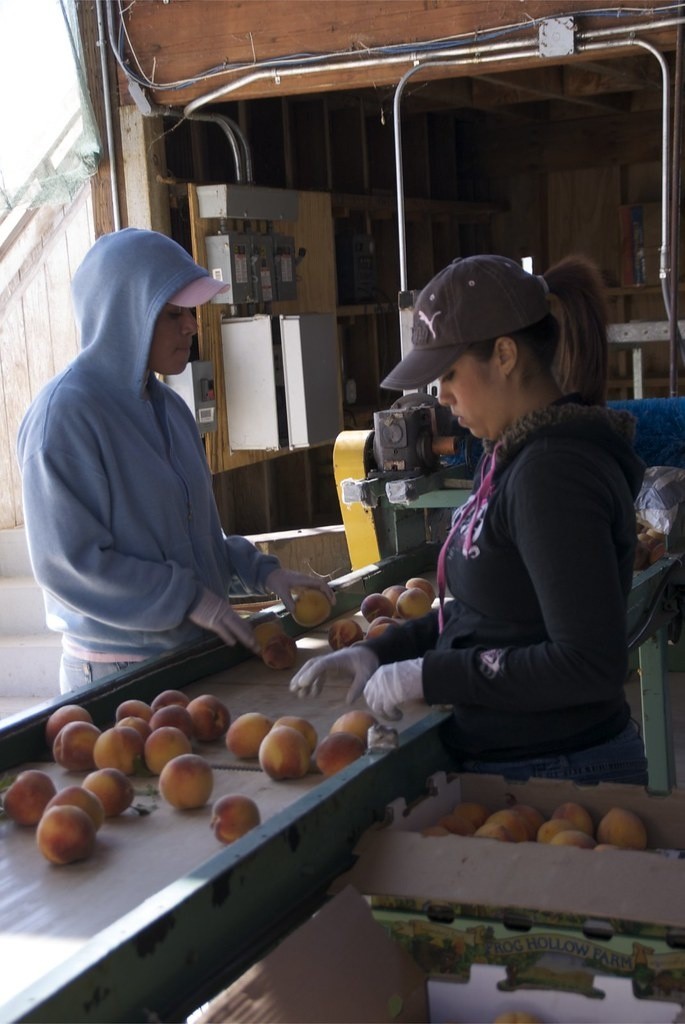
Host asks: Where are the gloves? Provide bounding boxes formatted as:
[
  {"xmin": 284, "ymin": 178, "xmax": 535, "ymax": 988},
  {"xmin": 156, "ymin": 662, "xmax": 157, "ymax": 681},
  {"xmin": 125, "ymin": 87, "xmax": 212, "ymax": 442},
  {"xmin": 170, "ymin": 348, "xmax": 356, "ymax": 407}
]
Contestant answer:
[
  {"xmin": 264, "ymin": 568, "xmax": 336, "ymax": 613},
  {"xmin": 363, "ymin": 657, "xmax": 425, "ymax": 721},
  {"xmin": 289, "ymin": 644, "xmax": 379, "ymax": 704},
  {"xmin": 187, "ymin": 586, "xmax": 262, "ymax": 654}
]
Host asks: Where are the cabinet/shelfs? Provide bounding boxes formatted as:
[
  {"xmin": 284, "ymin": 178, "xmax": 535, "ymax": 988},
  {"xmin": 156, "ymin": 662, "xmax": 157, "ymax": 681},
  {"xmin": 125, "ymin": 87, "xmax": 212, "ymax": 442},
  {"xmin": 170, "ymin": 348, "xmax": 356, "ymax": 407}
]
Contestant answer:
[{"xmin": 188, "ymin": 86, "xmax": 685, "ymax": 540}]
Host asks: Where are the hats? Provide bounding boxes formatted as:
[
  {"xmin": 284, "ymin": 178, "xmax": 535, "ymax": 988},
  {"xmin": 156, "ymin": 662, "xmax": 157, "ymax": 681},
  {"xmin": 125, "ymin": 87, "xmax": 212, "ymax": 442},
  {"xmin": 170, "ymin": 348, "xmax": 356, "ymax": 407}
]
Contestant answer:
[
  {"xmin": 166, "ymin": 276, "xmax": 231, "ymax": 307},
  {"xmin": 379, "ymin": 254, "xmax": 551, "ymax": 391}
]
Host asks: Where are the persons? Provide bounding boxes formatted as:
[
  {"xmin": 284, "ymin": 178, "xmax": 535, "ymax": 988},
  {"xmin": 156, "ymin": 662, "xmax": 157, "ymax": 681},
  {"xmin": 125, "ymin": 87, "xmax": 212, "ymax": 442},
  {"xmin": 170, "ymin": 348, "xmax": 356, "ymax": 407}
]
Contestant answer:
[
  {"xmin": 290, "ymin": 256, "xmax": 649, "ymax": 787},
  {"xmin": 15, "ymin": 227, "xmax": 338, "ymax": 696}
]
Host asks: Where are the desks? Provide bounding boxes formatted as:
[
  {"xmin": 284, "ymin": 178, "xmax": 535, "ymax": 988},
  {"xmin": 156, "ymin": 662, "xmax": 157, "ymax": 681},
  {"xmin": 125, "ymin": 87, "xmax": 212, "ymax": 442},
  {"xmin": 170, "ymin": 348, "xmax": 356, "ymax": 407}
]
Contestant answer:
[{"xmin": 0, "ymin": 541, "xmax": 685, "ymax": 1024}]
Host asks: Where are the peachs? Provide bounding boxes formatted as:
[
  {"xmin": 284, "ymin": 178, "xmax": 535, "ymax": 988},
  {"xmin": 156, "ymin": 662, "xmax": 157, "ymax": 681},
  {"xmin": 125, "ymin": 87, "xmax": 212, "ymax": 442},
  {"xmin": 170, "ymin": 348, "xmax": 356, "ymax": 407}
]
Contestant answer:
[
  {"xmin": 256, "ymin": 578, "xmax": 436, "ymax": 668},
  {"xmin": 491, "ymin": 1012, "xmax": 542, "ymax": 1024},
  {"xmin": 637, "ymin": 517, "xmax": 670, "ymax": 573},
  {"xmin": 0, "ymin": 690, "xmax": 380, "ymax": 866},
  {"xmin": 415, "ymin": 799, "xmax": 648, "ymax": 852}
]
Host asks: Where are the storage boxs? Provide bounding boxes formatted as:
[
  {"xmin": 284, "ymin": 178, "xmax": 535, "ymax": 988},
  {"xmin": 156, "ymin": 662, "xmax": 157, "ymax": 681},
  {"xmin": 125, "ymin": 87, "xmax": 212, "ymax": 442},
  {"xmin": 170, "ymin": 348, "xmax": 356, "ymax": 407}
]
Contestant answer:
[{"xmin": 324, "ymin": 770, "xmax": 685, "ymax": 1006}]
